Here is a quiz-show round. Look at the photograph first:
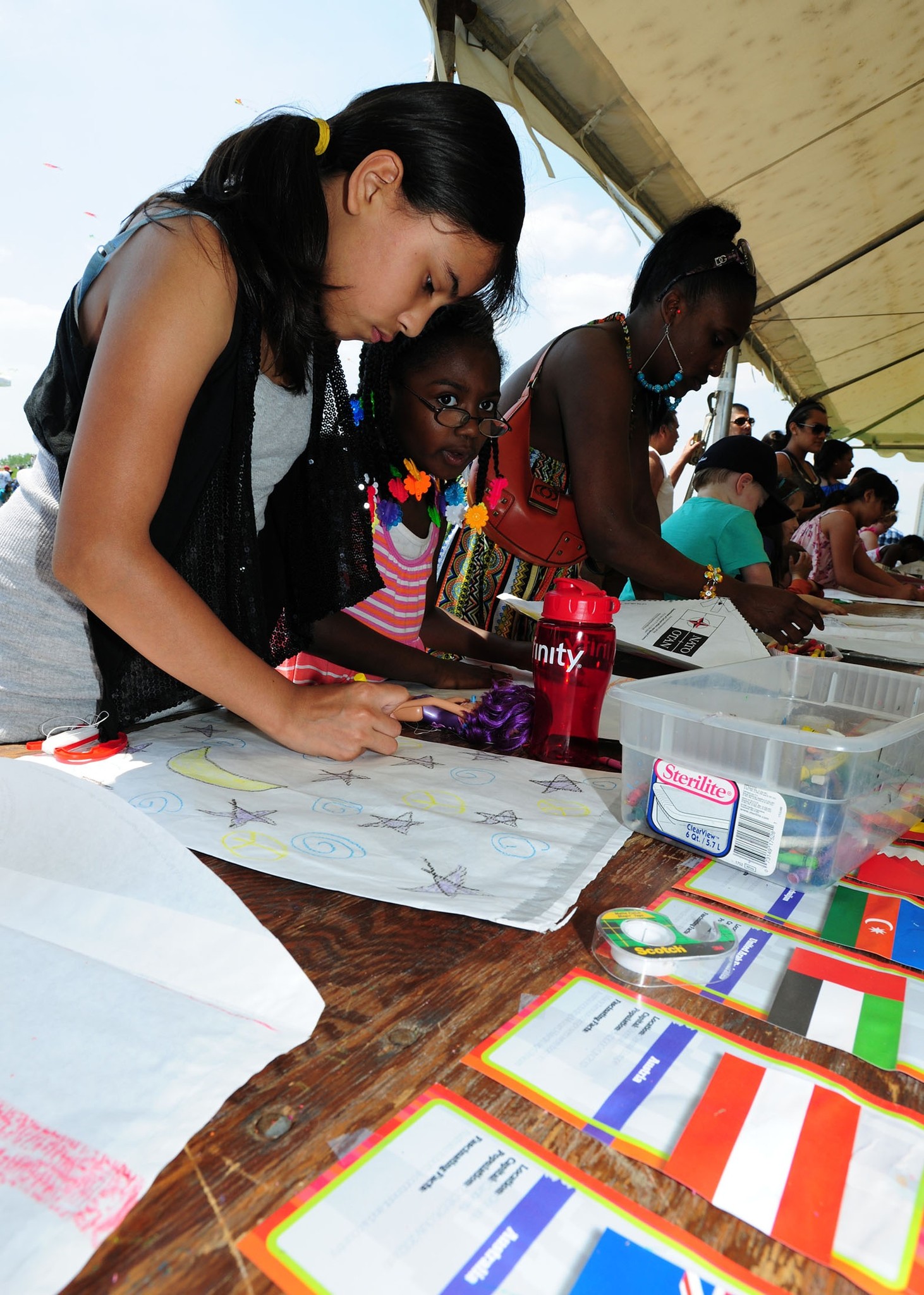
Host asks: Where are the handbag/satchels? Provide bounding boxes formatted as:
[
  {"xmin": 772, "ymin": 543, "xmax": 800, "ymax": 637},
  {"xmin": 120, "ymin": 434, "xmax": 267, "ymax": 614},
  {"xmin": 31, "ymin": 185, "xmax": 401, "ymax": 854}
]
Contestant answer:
[{"xmin": 434, "ymin": 325, "xmax": 587, "ymax": 642}]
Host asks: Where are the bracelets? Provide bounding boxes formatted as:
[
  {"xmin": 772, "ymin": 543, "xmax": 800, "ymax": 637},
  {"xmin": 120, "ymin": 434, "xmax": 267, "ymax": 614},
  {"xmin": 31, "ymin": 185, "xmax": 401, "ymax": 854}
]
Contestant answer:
[{"xmin": 700, "ymin": 565, "xmax": 724, "ymax": 600}]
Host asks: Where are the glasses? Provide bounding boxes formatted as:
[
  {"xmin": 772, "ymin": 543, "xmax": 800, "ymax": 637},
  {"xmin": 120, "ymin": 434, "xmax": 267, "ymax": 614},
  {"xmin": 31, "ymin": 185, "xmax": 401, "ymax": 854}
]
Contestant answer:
[
  {"xmin": 729, "ymin": 417, "xmax": 755, "ymax": 427},
  {"xmin": 655, "ymin": 239, "xmax": 756, "ymax": 304},
  {"xmin": 799, "ymin": 423, "xmax": 832, "ymax": 435},
  {"xmin": 409, "ymin": 387, "xmax": 514, "ymax": 439}
]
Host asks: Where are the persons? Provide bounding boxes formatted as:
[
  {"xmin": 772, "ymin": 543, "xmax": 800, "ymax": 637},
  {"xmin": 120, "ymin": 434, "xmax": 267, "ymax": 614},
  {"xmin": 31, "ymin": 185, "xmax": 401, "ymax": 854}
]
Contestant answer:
[
  {"xmin": 257, "ymin": 297, "xmax": 589, "ymax": 689},
  {"xmin": 647, "ymin": 400, "xmax": 924, "ymax": 616},
  {"xmin": 0, "ymin": 466, "xmax": 23, "ymax": 507},
  {"xmin": 435, "ymin": 203, "xmax": 825, "ymax": 645},
  {"xmin": 0, "ymin": 81, "xmax": 525, "ymax": 762}
]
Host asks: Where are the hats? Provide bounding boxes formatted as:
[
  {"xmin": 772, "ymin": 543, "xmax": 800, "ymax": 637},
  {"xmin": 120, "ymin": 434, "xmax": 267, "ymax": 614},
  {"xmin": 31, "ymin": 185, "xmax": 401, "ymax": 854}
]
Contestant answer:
[{"xmin": 694, "ymin": 435, "xmax": 797, "ymax": 533}]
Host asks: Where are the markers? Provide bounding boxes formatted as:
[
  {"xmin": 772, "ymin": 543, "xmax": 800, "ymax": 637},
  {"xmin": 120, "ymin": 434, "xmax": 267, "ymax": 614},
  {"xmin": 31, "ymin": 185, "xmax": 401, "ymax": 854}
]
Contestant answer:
[
  {"xmin": 354, "ymin": 673, "xmax": 366, "ymax": 682},
  {"xmin": 777, "ymin": 635, "xmax": 828, "ymax": 657},
  {"xmin": 627, "ymin": 725, "xmax": 908, "ymax": 884},
  {"xmin": 599, "ymin": 755, "xmax": 621, "ymax": 770}
]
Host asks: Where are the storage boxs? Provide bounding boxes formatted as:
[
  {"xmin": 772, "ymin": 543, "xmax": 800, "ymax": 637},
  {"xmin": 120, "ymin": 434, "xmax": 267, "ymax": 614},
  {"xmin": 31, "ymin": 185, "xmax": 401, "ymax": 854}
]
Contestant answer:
[{"xmin": 603, "ymin": 653, "xmax": 923, "ymax": 890}]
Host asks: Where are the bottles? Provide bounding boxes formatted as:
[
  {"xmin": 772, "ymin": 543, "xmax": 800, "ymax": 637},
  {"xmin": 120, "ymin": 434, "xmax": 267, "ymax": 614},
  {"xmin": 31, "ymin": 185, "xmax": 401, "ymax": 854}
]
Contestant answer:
[{"xmin": 533, "ymin": 578, "xmax": 620, "ymax": 764}]
